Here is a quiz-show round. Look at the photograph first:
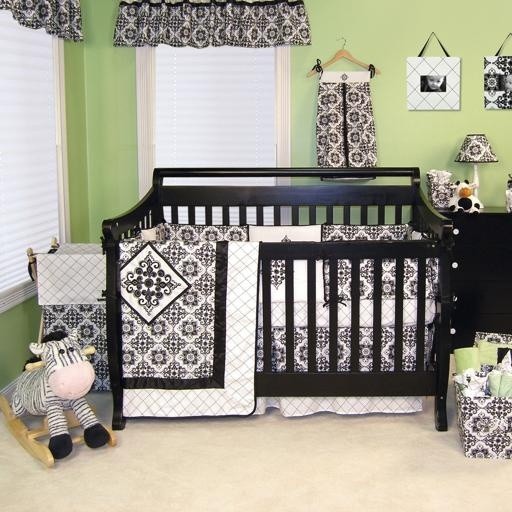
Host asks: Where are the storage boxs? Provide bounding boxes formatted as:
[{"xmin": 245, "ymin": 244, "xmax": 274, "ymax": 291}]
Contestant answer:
[{"xmin": 452, "ymin": 332, "xmax": 511, "ymax": 460}]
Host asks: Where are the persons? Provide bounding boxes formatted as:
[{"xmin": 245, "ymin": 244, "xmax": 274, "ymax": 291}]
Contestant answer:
[{"xmin": 421, "ymin": 76, "xmax": 446, "ymax": 92}]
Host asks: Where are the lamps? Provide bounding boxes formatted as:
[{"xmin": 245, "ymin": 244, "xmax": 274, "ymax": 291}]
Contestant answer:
[{"xmin": 454, "ymin": 133, "xmax": 498, "ymax": 208}]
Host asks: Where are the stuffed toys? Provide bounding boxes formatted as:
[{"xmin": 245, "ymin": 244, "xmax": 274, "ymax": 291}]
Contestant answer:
[{"xmin": 446, "ymin": 180, "xmax": 484, "ymax": 215}]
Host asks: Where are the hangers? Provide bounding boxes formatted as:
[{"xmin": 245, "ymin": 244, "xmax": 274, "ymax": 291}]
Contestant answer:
[{"xmin": 305, "ymin": 36, "xmax": 383, "ymax": 80}]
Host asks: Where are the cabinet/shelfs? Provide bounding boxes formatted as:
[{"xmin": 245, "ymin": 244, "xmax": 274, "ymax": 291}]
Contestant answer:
[{"xmin": 437, "ymin": 206, "xmax": 511, "ymax": 353}]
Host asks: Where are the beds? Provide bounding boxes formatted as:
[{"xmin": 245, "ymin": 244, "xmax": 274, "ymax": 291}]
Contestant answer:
[{"xmin": 100, "ymin": 166, "xmax": 453, "ymax": 431}]
